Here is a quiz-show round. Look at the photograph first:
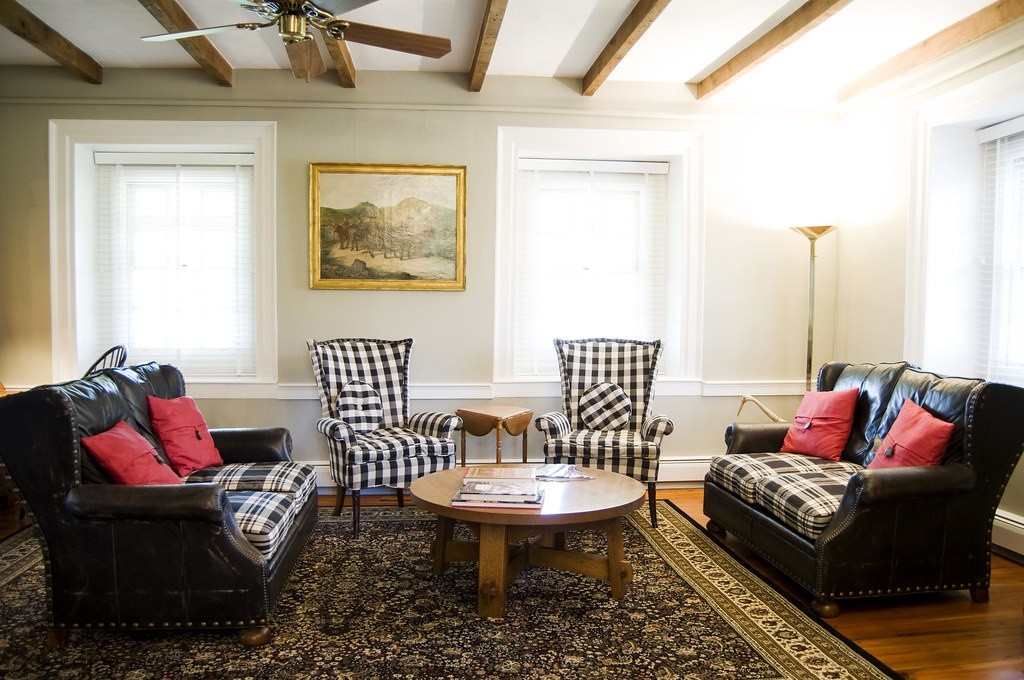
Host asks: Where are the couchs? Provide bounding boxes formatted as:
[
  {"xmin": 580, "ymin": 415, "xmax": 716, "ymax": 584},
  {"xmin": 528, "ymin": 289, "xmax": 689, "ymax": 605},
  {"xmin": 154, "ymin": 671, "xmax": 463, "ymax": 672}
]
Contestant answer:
[
  {"xmin": 704, "ymin": 362, "xmax": 1024, "ymax": 618},
  {"xmin": 0, "ymin": 362, "xmax": 319, "ymax": 647}
]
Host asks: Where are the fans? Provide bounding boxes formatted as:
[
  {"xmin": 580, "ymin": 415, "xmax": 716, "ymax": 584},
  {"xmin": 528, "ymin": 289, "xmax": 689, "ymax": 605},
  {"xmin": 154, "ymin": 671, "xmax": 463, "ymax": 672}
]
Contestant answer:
[{"xmin": 140, "ymin": 0, "xmax": 452, "ymax": 60}]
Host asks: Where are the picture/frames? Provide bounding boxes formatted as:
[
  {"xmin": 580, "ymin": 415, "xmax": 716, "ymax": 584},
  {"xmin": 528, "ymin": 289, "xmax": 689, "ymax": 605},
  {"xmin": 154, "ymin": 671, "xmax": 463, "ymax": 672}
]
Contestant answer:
[{"xmin": 308, "ymin": 162, "xmax": 467, "ymax": 291}]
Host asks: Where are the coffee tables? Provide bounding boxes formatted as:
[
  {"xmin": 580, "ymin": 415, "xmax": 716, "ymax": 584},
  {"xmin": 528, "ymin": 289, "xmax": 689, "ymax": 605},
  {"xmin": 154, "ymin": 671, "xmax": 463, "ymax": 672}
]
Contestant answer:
[{"xmin": 410, "ymin": 463, "xmax": 645, "ymax": 618}]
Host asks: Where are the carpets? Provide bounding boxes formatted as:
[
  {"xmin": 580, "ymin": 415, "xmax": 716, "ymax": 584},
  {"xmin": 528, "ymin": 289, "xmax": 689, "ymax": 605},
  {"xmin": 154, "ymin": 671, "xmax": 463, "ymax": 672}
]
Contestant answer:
[{"xmin": 1, "ymin": 499, "xmax": 904, "ymax": 680}]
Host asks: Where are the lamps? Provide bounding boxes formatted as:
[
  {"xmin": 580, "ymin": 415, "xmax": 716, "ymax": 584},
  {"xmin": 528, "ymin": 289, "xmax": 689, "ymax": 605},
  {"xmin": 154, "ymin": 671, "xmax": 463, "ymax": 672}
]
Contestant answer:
[{"xmin": 790, "ymin": 224, "xmax": 836, "ymax": 392}]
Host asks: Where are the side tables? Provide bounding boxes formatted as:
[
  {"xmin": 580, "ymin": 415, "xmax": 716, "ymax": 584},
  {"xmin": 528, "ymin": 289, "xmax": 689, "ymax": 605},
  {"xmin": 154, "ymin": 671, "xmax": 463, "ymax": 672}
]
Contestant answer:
[{"xmin": 455, "ymin": 405, "xmax": 534, "ymax": 467}]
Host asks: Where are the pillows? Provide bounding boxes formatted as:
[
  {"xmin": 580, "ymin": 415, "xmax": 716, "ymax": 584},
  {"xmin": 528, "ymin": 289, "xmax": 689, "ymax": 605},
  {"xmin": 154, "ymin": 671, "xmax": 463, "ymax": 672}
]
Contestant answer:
[
  {"xmin": 579, "ymin": 382, "xmax": 633, "ymax": 432},
  {"xmin": 781, "ymin": 388, "xmax": 858, "ymax": 462},
  {"xmin": 81, "ymin": 421, "xmax": 182, "ymax": 486},
  {"xmin": 336, "ymin": 380, "xmax": 383, "ymax": 434},
  {"xmin": 148, "ymin": 394, "xmax": 223, "ymax": 477},
  {"xmin": 867, "ymin": 399, "xmax": 955, "ymax": 469}
]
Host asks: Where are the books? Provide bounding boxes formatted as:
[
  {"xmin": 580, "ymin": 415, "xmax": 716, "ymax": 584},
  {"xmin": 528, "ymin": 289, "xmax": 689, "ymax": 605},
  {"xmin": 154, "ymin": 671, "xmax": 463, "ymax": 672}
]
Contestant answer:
[
  {"xmin": 450, "ymin": 468, "xmax": 546, "ymax": 509},
  {"xmin": 536, "ymin": 464, "xmax": 595, "ymax": 482}
]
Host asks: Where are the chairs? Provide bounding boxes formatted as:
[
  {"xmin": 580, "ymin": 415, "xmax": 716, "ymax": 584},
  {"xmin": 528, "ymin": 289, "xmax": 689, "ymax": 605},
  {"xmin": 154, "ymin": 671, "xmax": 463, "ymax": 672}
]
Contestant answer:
[
  {"xmin": 84, "ymin": 346, "xmax": 127, "ymax": 376},
  {"xmin": 535, "ymin": 338, "xmax": 674, "ymax": 528},
  {"xmin": 306, "ymin": 337, "xmax": 464, "ymax": 540}
]
